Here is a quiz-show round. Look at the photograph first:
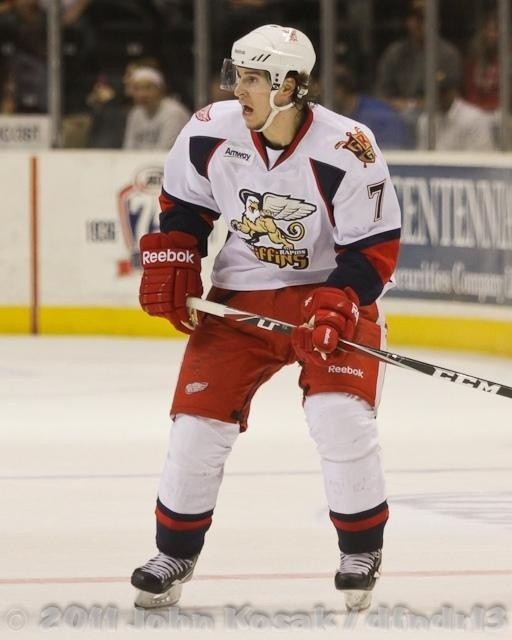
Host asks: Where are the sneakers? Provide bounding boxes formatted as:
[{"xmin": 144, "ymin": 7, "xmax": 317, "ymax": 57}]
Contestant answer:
[
  {"xmin": 131, "ymin": 551, "xmax": 198, "ymax": 594},
  {"xmin": 335, "ymin": 549, "xmax": 382, "ymax": 591}
]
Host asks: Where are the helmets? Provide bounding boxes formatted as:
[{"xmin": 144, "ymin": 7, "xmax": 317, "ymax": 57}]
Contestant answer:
[{"xmin": 219, "ymin": 23, "xmax": 316, "ymax": 95}]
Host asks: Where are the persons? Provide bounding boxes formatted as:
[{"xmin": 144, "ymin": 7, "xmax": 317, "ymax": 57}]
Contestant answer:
[
  {"xmin": 129, "ymin": 22, "xmax": 402, "ymax": 594},
  {"xmin": 0, "ymin": 0, "xmax": 512, "ymax": 153}
]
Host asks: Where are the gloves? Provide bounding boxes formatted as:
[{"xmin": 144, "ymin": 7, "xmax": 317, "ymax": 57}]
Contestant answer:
[
  {"xmin": 291, "ymin": 285, "xmax": 360, "ymax": 368},
  {"xmin": 136, "ymin": 230, "xmax": 203, "ymax": 335}
]
